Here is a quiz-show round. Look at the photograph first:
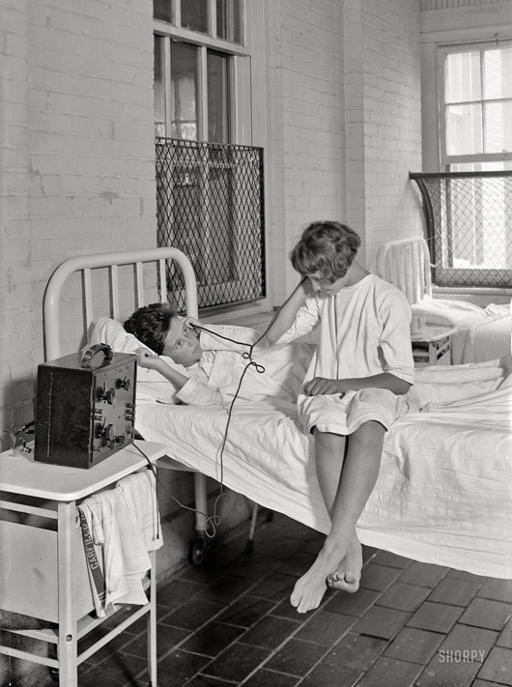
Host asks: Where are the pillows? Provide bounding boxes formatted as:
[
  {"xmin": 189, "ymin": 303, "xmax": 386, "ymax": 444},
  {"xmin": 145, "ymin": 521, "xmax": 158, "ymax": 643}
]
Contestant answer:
[{"xmin": 410, "ymin": 293, "xmax": 469, "ymax": 327}]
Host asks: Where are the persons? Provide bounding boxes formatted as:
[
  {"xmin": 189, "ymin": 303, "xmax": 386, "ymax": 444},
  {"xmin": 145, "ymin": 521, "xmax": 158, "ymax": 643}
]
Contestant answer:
[
  {"xmin": 265, "ymin": 219, "xmax": 417, "ymax": 618},
  {"xmin": 123, "ymin": 298, "xmax": 510, "ymax": 416}
]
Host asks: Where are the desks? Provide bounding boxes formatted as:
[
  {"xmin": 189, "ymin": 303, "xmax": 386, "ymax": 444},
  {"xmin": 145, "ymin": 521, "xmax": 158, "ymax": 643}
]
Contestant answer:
[
  {"xmin": 0, "ymin": 435, "xmax": 169, "ymax": 674},
  {"xmin": 410, "ymin": 325, "xmax": 458, "ymax": 366}
]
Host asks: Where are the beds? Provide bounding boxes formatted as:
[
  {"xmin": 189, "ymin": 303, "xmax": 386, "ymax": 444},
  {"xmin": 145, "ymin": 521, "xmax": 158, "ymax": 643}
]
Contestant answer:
[
  {"xmin": 42, "ymin": 245, "xmax": 512, "ymax": 581},
  {"xmin": 378, "ymin": 237, "xmax": 511, "ymax": 365}
]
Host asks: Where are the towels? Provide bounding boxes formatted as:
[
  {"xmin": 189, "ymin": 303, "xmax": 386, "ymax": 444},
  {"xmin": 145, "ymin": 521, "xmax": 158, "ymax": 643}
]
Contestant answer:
[{"xmin": 77, "ymin": 468, "xmax": 165, "ymax": 617}]
